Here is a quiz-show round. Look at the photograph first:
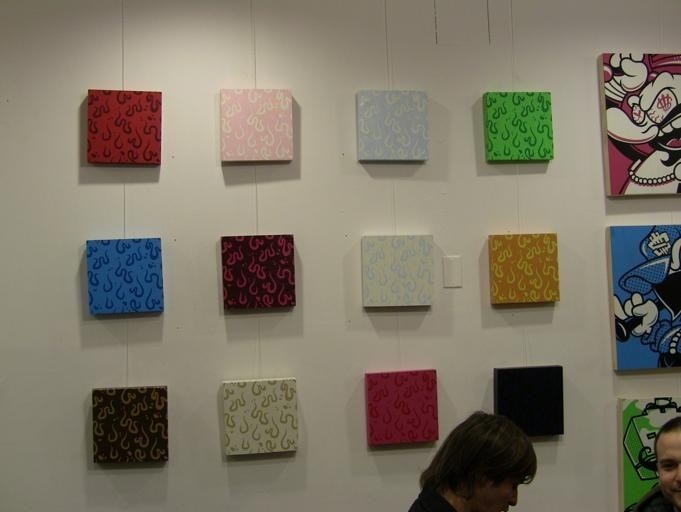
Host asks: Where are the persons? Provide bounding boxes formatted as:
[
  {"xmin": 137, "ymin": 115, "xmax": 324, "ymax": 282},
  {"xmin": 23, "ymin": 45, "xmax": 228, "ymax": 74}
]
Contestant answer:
[
  {"xmin": 407, "ymin": 409, "xmax": 538, "ymax": 512},
  {"xmin": 623, "ymin": 416, "xmax": 681, "ymax": 512}
]
